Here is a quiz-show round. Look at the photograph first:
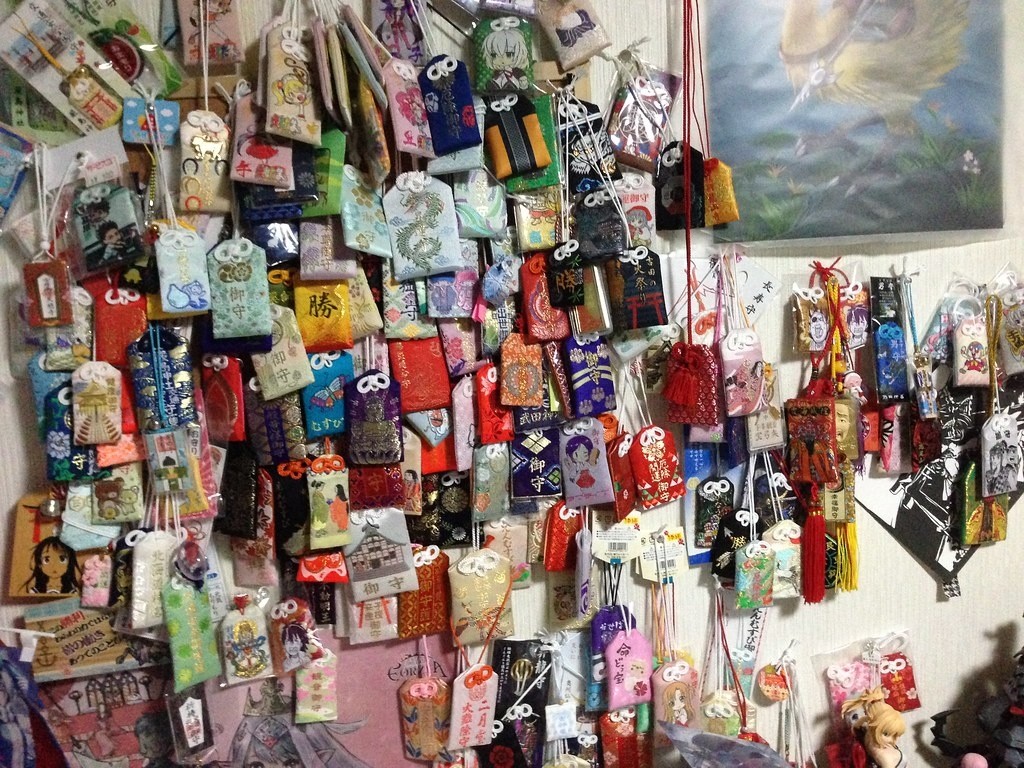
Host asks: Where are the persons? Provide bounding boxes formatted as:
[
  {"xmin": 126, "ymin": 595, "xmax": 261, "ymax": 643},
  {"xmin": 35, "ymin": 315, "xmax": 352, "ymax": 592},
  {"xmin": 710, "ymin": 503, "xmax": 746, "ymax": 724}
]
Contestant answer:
[{"xmin": 841, "ymin": 684, "xmax": 913, "ymax": 768}]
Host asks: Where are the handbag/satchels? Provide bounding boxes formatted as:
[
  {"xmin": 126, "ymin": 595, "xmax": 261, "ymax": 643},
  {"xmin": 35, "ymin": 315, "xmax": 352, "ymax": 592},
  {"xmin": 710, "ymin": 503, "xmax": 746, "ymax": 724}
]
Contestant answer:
[{"xmin": 4, "ymin": 0, "xmax": 1024, "ymax": 768}]
